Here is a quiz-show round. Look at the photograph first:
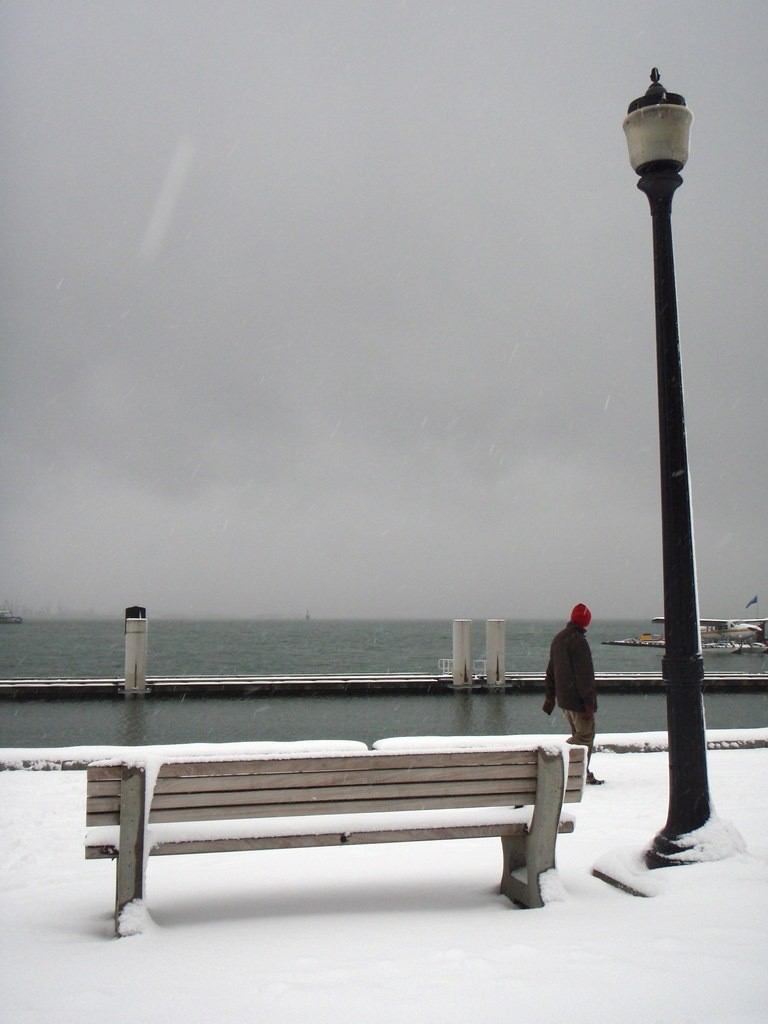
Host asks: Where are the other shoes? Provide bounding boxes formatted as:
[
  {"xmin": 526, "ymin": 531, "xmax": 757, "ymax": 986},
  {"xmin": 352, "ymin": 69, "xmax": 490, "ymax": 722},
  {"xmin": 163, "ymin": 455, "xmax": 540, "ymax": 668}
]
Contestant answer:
[{"xmin": 586, "ymin": 772, "xmax": 604, "ymax": 785}]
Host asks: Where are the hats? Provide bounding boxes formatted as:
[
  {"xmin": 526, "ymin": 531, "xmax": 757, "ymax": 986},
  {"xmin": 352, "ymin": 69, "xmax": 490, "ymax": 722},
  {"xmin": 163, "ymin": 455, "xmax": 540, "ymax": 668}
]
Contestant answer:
[{"xmin": 571, "ymin": 603, "xmax": 592, "ymax": 627}]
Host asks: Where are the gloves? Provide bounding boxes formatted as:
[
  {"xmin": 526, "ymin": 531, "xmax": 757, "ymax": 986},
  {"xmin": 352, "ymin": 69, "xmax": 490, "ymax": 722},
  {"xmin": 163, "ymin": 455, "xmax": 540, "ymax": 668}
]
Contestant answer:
[
  {"xmin": 543, "ymin": 699, "xmax": 556, "ymax": 716},
  {"xmin": 582, "ymin": 705, "xmax": 594, "ymax": 721}
]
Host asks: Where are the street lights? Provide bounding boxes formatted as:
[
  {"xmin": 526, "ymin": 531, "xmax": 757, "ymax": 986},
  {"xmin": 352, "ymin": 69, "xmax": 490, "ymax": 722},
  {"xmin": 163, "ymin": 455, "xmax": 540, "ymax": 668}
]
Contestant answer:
[{"xmin": 622, "ymin": 64, "xmax": 751, "ymax": 870}]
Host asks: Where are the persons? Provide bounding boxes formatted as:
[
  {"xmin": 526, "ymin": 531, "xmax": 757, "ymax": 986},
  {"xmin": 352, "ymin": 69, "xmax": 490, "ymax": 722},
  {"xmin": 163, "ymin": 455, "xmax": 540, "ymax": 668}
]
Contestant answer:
[{"xmin": 542, "ymin": 602, "xmax": 606, "ymax": 785}]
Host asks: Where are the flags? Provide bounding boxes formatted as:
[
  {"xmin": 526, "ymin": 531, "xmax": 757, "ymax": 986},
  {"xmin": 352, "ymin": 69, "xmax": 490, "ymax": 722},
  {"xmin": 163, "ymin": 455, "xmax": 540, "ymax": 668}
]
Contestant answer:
[{"xmin": 746, "ymin": 594, "xmax": 757, "ymax": 608}]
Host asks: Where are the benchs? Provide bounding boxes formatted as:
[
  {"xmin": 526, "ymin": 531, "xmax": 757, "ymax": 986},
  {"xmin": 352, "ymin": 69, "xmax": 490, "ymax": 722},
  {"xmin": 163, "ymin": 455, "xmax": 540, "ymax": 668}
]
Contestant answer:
[{"xmin": 85, "ymin": 744, "xmax": 585, "ymax": 936}]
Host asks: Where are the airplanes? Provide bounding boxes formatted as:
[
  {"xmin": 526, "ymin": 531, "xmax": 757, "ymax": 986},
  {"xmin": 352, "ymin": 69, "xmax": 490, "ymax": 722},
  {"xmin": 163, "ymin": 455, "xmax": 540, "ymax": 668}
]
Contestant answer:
[{"xmin": 651, "ymin": 616, "xmax": 768, "ymax": 656}]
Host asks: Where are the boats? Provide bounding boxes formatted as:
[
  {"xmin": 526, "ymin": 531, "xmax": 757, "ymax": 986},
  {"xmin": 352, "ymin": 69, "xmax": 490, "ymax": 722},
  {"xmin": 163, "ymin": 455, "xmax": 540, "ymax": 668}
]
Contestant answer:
[{"xmin": 0, "ymin": 601, "xmax": 24, "ymax": 625}]
